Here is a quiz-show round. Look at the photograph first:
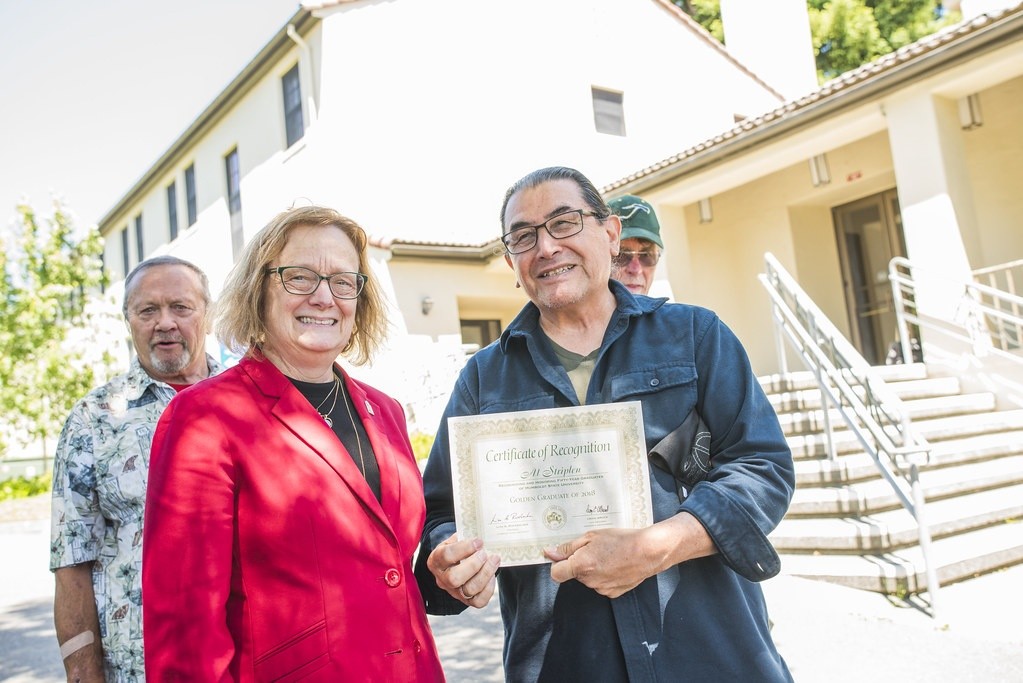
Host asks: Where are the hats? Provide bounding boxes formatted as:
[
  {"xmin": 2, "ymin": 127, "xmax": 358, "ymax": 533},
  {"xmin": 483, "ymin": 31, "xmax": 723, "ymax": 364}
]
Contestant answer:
[
  {"xmin": 606, "ymin": 193, "xmax": 664, "ymax": 249},
  {"xmin": 648, "ymin": 410, "xmax": 711, "ymax": 504}
]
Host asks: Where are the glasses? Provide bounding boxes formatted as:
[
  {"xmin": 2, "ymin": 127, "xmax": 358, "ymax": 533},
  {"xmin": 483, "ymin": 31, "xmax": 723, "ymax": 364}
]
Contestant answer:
[
  {"xmin": 500, "ymin": 209, "xmax": 598, "ymax": 254},
  {"xmin": 612, "ymin": 252, "xmax": 661, "ymax": 267},
  {"xmin": 265, "ymin": 266, "xmax": 367, "ymax": 299}
]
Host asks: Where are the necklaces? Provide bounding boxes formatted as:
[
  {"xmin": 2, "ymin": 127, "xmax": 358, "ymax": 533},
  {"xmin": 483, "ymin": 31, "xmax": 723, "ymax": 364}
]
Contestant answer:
[{"xmin": 317, "ymin": 378, "xmax": 340, "ymax": 428}]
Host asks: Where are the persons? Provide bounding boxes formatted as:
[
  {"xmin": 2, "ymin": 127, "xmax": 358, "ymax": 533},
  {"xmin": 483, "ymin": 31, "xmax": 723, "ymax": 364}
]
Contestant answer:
[
  {"xmin": 141, "ymin": 204, "xmax": 445, "ymax": 683},
  {"xmin": 412, "ymin": 165, "xmax": 796, "ymax": 683},
  {"xmin": 606, "ymin": 192, "xmax": 663, "ymax": 298},
  {"xmin": 49, "ymin": 253, "xmax": 229, "ymax": 683}
]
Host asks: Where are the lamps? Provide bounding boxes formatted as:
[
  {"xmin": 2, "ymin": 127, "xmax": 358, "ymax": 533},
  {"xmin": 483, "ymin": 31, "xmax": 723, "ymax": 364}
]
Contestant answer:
[
  {"xmin": 421, "ymin": 296, "xmax": 434, "ymax": 316},
  {"xmin": 698, "ymin": 198, "xmax": 713, "ymax": 224},
  {"xmin": 959, "ymin": 93, "xmax": 985, "ymax": 133},
  {"xmin": 810, "ymin": 153, "xmax": 830, "ymax": 188}
]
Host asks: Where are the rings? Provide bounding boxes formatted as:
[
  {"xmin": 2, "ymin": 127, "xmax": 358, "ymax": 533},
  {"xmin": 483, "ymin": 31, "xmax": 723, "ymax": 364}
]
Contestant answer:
[{"xmin": 459, "ymin": 586, "xmax": 473, "ymax": 600}]
